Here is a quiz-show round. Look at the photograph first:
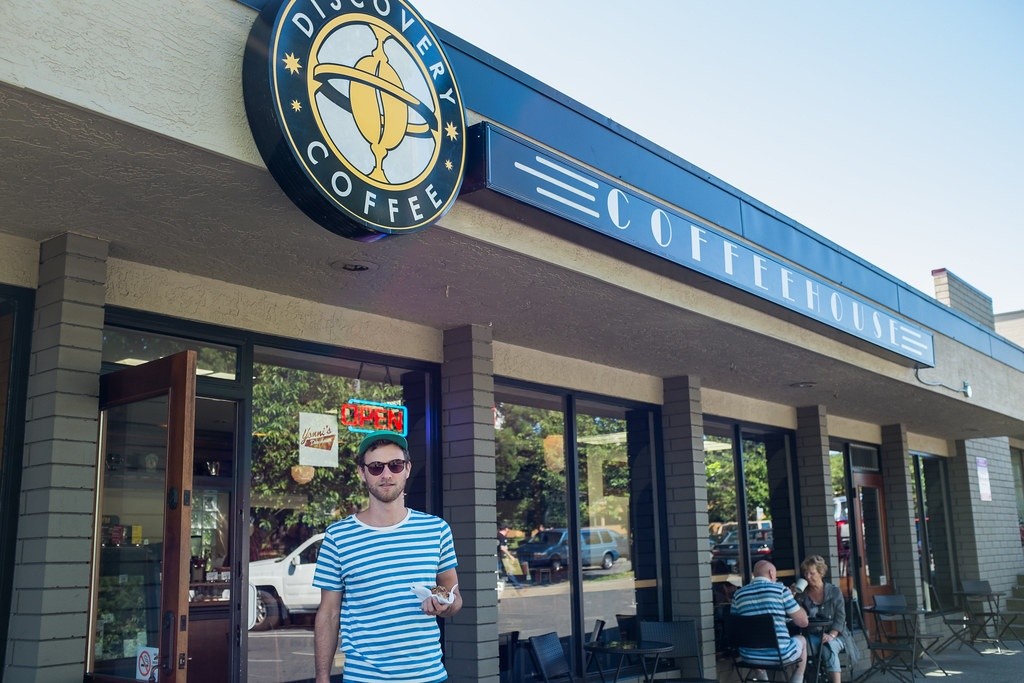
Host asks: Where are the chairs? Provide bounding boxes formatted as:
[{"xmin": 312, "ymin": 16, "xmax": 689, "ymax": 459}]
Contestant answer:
[
  {"xmin": 808, "ymin": 599, "xmax": 856, "ymax": 683},
  {"xmin": 958, "ymin": 580, "xmax": 1024, "ymax": 650},
  {"xmin": 638, "ymin": 619, "xmax": 721, "ymax": 683},
  {"xmin": 873, "ymin": 594, "xmax": 949, "ymax": 678},
  {"xmin": 929, "ymin": 585, "xmax": 987, "ymax": 658},
  {"xmin": 529, "ymin": 631, "xmax": 575, "ymax": 683},
  {"xmin": 584, "ymin": 619, "xmax": 607, "ymax": 683},
  {"xmin": 851, "ymin": 598, "xmax": 917, "ymax": 683},
  {"xmin": 614, "ymin": 613, "xmax": 650, "ymax": 682},
  {"xmin": 721, "ymin": 612, "xmax": 803, "ymax": 683}
]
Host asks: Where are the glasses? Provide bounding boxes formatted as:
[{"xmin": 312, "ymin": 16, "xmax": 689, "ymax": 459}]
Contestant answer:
[{"xmin": 362, "ymin": 459, "xmax": 408, "ymax": 476}]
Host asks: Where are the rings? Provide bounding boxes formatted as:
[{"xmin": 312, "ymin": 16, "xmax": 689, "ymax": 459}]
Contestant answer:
[{"xmin": 826, "ymin": 638, "xmax": 827, "ymax": 640}]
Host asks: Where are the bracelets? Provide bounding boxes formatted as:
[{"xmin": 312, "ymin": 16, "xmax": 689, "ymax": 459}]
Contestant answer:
[{"xmin": 830, "ymin": 633, "xmax": 834, "ymax": 638}]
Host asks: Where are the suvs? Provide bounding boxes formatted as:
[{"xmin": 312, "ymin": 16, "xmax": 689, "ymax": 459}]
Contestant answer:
[
  {"xmin": 516, "ymin": 526, "xmax": 621, "ymax": 572},
  {"xmin": 247, "ymin": 533, "xmax": 325, "ymax": 631}
]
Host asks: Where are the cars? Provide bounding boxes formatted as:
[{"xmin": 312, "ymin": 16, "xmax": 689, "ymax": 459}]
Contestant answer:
[{"xmin": 711, "ymin": 526, "xmax": 775, "ymax": 569}]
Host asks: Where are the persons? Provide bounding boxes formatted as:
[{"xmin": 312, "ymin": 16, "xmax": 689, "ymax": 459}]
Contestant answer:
[
  {"xmin": 790, "ymin": 555, "xmax": 856, "ymax": 683},
  {"xmin": 731, "ymin": 560, "xmax": 809, "ymax": 683},
  {"xmin": 498, "ymin": 525, "xmax": 522, "ymax": 588},
  {"xmin": 312, "ymin": 430, "xmax": 462, "ymax": 683}
]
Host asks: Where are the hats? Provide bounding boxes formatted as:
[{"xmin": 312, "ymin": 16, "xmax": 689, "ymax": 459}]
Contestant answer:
[
  {"xmin": 500, "ymin": 524, "xmax": 512, "ymax": 530},
  {"xmin": 358, "ymin": 429, "xmax": 408, "ymax": 457}
]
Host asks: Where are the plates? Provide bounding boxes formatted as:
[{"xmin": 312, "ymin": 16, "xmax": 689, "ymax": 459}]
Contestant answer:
[{"xmin": 145, "ymin": 453, "xmax": 159, "ymax": 469}]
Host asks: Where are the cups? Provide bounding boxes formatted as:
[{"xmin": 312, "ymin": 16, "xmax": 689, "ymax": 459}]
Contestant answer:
[{"xmin": 794, "ymin": 578, "xmax": 808, "ymax": 593}]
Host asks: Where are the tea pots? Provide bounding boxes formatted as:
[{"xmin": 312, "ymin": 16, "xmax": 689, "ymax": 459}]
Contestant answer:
[{"xmin": 207, "ymin": 461, "xmax": 220, "ymax": 476}]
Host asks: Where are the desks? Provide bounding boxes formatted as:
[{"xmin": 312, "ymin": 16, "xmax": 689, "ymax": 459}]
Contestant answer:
[
  {"xmin": 947, "ymin": 589, "xmax": 1007, "ymax": 653},
  {"xmin": 860, "ymin": 604, "xmax": 936, "ymax": 680},
  {"xmin": 582, "ymin": 639, "xmax": 676, "ymax": 683},
  {"xmin": 785, "ymin": 616, "xmax": 834, "ymax": 683}
]
